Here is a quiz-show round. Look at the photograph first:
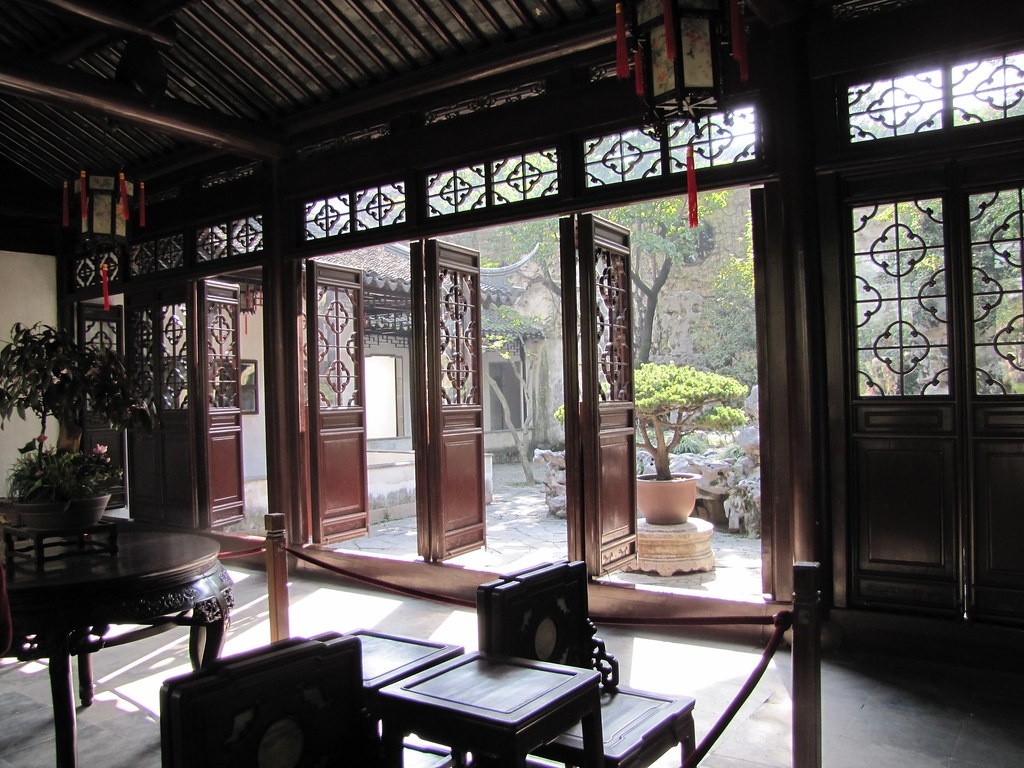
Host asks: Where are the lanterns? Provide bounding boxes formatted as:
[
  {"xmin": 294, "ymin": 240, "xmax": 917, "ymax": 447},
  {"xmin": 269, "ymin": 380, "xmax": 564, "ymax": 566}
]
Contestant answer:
[
  {"xmin": 634, "ymin": 0, "xmax": 728, "ymax": 229},
  {"xmin": 64, "ymin": 169, "xmax": 146, "ymax": 309}
]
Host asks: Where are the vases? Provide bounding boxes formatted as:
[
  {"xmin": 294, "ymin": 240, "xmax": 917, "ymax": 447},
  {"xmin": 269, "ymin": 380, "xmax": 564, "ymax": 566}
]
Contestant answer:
[{"xmin": 12, "ymin": 494, "xmax": 112, "ymax": 528}]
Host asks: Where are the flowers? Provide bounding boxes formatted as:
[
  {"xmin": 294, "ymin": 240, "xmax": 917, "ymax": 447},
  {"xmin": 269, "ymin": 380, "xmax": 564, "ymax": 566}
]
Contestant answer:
[{"xmin": 11, "ymin": 435, "xmax": 124, "ymax": 529}]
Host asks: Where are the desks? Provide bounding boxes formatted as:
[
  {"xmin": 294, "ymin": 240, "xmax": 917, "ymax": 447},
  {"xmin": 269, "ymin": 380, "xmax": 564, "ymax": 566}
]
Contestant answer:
[
  {"xmin": 378, "ymin": 650, "xmax": 606, "ymax": 768},
  {"xmin": 0, "ymin": 531, "xmax": 235, "ymax": 768},
  {"xmin": 343, "ymin": 628, "xmax": 466, "ymax": 704}
]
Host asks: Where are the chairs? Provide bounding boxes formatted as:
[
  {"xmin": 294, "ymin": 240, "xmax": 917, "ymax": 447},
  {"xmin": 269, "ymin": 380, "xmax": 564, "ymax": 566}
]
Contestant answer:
[
  {"xmin": 160, "ymin": 631, "xmax": 369, "ymax": 768},
  {"xmin": 478, "ymin": 558, "xmax": 696, "ymax": 768}
]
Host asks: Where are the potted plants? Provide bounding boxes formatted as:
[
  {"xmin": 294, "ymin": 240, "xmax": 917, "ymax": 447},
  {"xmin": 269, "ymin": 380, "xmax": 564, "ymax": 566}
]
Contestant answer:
[{"xmin": 555, "ymin": 360, "xmax": 751, "ymax": 526}]
